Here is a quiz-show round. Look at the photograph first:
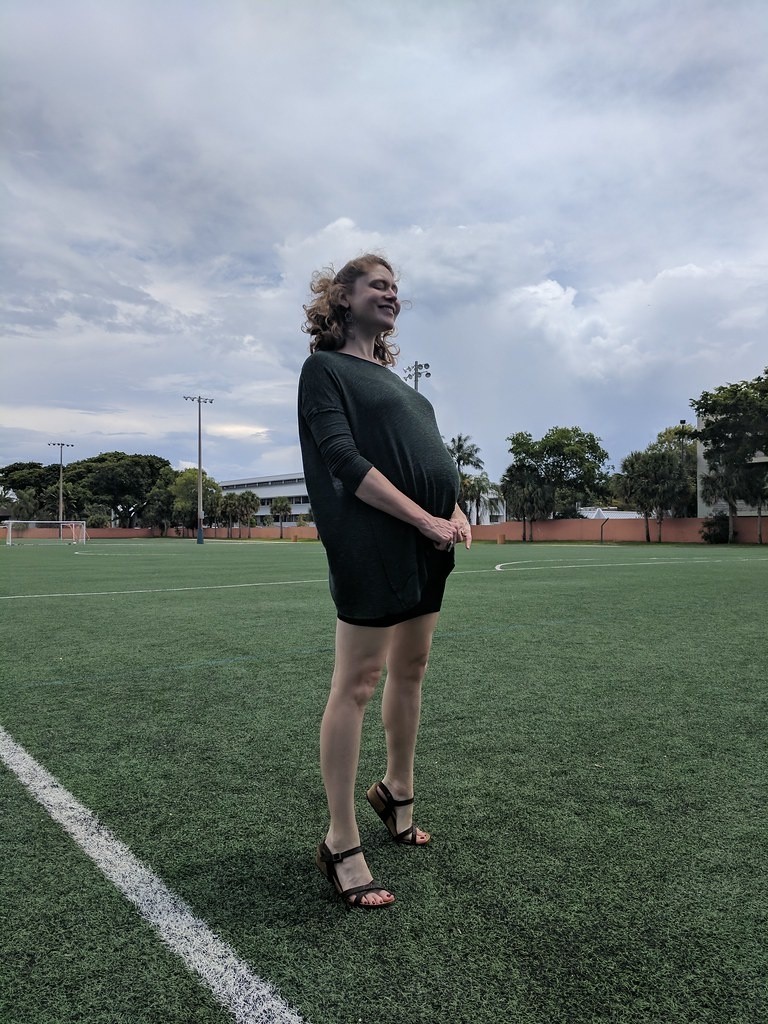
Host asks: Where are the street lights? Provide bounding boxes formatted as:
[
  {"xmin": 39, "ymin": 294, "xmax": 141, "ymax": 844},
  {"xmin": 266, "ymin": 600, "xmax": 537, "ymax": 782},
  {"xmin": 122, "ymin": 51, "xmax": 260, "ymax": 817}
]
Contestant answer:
[
  {"xmin": 182, "ymin": 395, "xmax": 215, "ymax": 544},
  {"xmin": 47, "ymin": 442, "xmax": 75, "ymax": 539},
  {"xmin": 402, "ymin": 360, "xmax": 432, "ymax": 392}
]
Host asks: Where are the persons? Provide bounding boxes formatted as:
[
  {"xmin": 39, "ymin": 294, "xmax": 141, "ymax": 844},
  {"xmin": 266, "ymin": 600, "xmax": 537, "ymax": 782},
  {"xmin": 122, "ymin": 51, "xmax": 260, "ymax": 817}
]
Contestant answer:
[{"xmin": 297, "ymin": 255, "xmax": 472, "ymax": 908}]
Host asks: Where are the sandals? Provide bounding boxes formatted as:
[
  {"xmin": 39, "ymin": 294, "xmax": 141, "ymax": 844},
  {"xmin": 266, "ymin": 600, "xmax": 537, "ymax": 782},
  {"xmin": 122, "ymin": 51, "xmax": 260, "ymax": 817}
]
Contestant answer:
[
  {"xmin": 366, "ymin": 781, "xmax": 431, "ymax": 846},
  {"xmin": 315, "ymin": 839, "xmax": 395, "ymax": 908}
]
